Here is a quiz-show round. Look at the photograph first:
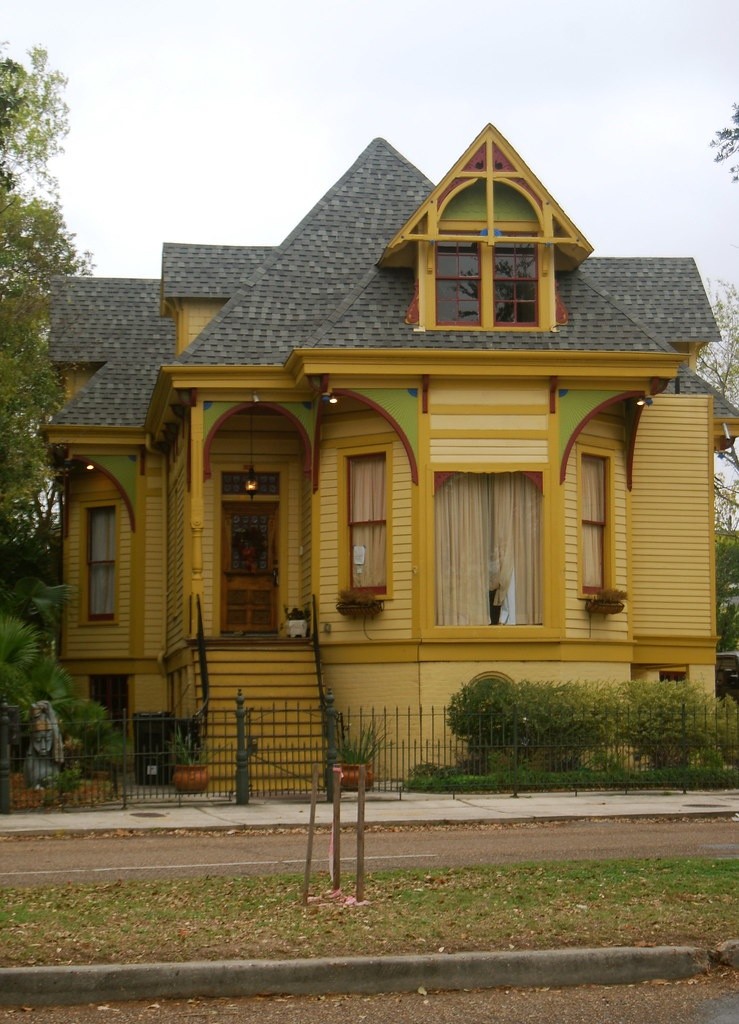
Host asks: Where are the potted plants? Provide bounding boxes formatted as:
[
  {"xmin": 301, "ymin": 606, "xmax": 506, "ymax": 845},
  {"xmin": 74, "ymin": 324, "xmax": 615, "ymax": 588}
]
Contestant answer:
[
  {"xmin": 284, "ymin": 606, "xmax": 307, "ymax": 638},
  {"xmin": 314, "ymin": 709, "xmax": 396, "ymax": 792},
  {"xmin": 334, "ymin": 585, "xmax": 382, "ymax": 618},
  {"xmin": 164, "ymin": 722, "xmax": 229, "ymax": 794},
  {"xmin": 585, "ymin": 586, "xmax": 628, "ymax": 615}
]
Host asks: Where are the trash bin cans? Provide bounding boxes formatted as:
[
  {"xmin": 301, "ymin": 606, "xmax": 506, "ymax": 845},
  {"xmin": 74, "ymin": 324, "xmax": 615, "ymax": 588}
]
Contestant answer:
[{"xmin": 132, "ymin": 711, "xmax": 177, "ymax": 785}]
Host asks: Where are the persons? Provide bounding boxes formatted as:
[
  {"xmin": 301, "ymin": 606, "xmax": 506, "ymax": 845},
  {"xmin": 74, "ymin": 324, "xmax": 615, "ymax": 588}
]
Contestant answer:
[{"xmin": 19, "ymin": 700, "xmax": 65, "ymax": 793}]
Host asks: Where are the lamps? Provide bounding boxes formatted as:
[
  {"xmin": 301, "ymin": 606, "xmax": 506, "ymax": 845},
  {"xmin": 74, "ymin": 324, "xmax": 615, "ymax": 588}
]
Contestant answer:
[
  {"xmin": 329, "ymin": 393, "xmax": 338, "ymax": 404},
  {"xmin": 244, "ymin": 410, "xmax": 258, "ymax": 501},
  {"xmin": 636, "ymin": 395, "xmax": 645, "ymax": 406}
]
